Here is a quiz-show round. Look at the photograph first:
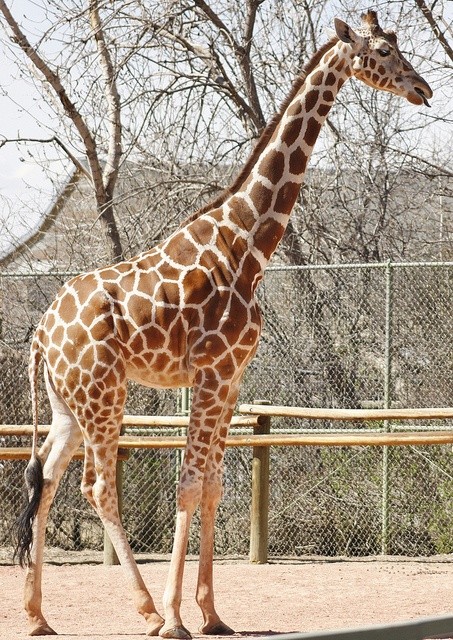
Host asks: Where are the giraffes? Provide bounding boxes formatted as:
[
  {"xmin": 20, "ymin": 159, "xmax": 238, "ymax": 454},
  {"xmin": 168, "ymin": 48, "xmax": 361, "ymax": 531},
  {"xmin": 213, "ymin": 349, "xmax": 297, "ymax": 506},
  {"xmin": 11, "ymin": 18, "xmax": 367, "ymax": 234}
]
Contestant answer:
[{"xmin": 11, "ymin": 9, "xmax": 433, "ymax": 640}]
